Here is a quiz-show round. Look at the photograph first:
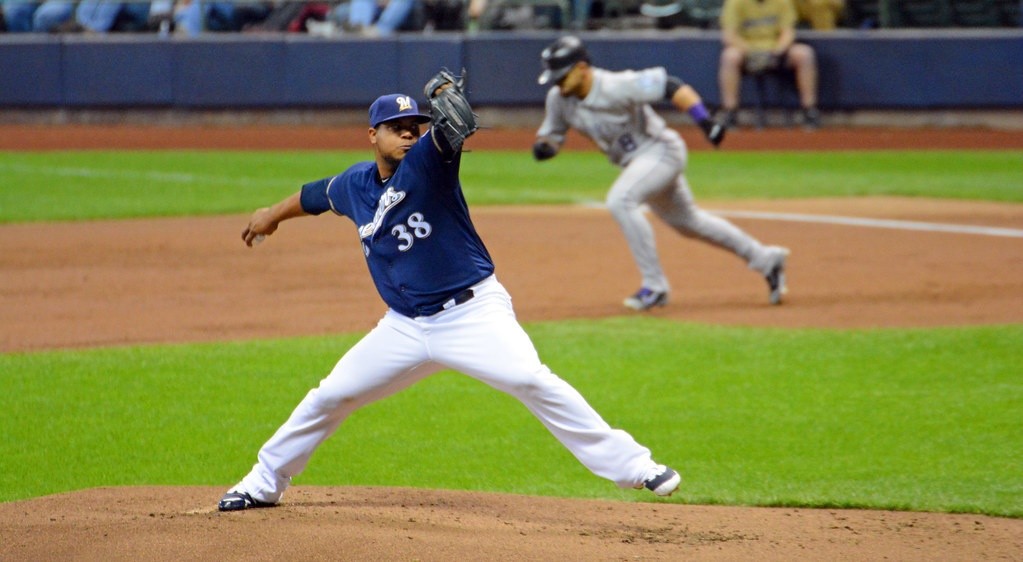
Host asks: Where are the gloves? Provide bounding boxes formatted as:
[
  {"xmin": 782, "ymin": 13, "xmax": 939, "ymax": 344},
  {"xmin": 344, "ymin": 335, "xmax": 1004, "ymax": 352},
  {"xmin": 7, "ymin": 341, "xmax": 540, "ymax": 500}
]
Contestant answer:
[
  {"xmin": 533, "ymin": 140, "xmax": 558, "ymax": 158},
  {"xmin": 702, "ymin": 118, "xmax": 726, "ymax": 144}
]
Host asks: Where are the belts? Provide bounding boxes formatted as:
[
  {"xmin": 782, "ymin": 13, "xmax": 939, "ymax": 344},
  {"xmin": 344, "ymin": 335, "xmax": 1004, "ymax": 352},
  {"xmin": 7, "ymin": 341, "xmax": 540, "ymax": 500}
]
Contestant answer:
[{"xmin": 431, "ymin": 289, "xmax": 473, "ymax": 313}]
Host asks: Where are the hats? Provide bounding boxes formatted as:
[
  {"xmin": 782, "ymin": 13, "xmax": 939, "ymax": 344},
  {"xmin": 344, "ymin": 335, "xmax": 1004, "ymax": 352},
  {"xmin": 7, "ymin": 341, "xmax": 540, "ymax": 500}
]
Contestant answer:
[
  {"xmin": 538, "ymin": 35, "xmax": 588, "ymax": 86},
  {"xmin": 367, "ymin": 93, "xmax": 432, "ymax": 128}
]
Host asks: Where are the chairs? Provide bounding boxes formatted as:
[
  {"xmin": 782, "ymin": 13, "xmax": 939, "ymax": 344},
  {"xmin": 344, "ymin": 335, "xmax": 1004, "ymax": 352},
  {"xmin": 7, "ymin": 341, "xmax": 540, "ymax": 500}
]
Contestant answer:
[{"xmin": 738, "ymin": 67, "xmax": 798, "ymax": 130}]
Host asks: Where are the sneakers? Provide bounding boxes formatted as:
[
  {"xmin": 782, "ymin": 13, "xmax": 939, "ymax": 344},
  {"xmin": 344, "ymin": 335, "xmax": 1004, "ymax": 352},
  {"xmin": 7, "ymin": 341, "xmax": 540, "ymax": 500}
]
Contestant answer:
[
  {"xmin": 218, "ymin": 493, "xmax": 272, "ymax": 510},
  {"xmin": 644, "ymin": 463, "xmax": 680, "ymax": 497},
  {"xmin": 623, "ymin": 288, "xmax": 667, "ymax": 311},
  {"xmin": 764, "ymin": 245, "xmax": 790, "ymax": 303}
]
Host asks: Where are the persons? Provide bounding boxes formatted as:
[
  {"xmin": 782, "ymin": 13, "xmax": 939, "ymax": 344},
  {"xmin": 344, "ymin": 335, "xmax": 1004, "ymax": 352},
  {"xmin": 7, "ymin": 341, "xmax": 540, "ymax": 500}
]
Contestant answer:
[
  {"xmin": 146, "ymin": 0, "xmax": 713, "ymax": 34},
  {"xmin": 217, "ymin": 71, "xmax": 681, "ymax": 511},
  {"xmin": 532, "ymin": 36, "xmax": 790, "ymax": 310},
  {"xmin": 718, "ymin": 0, "xmax": 820, "ymax": 129}
]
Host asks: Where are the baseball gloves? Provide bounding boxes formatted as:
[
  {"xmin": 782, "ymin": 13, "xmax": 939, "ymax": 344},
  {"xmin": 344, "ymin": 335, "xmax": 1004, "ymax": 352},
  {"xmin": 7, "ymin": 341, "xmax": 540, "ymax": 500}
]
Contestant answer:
[{"xmin": 422, "ymin": 73, "xmax": 479, "ymax": 152}]
